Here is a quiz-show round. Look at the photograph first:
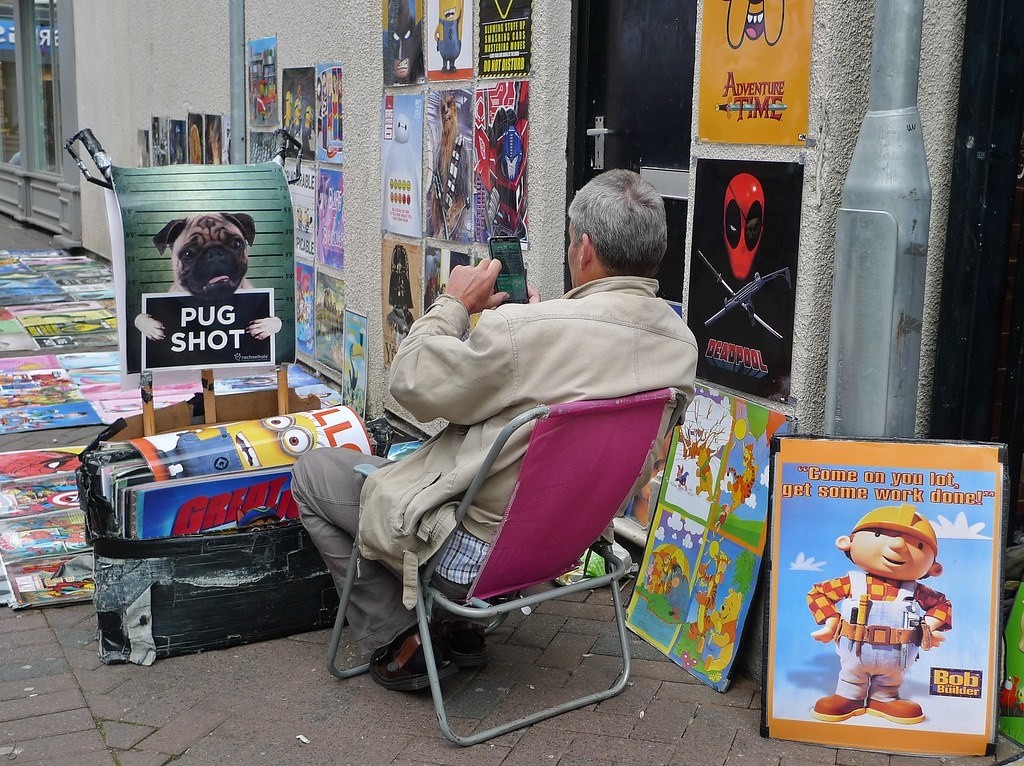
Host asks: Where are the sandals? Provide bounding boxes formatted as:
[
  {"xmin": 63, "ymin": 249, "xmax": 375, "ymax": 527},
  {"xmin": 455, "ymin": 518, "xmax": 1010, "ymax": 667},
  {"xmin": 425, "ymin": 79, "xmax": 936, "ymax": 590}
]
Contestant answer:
[
  {"xmin": 369, "ymin": 631, "xmax": 460, "ymax": 691},
  {"xmin": 429, "ymin": 619, "xmax": 489, "ymax": 666}
]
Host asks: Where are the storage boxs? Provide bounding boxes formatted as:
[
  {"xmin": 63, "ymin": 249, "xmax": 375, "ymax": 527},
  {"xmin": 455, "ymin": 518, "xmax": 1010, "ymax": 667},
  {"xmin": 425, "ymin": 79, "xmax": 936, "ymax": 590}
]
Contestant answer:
[{"xmin": 74, "ymin": 386, "xmax": 392, "ymax": 667}]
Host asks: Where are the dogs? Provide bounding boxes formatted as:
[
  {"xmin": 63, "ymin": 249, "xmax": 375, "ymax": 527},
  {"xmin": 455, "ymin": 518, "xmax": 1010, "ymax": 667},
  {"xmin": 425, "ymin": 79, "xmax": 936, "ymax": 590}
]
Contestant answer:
[{"xmin": 134, "ymin": 209, "xmax": 283, "ymax": 343}]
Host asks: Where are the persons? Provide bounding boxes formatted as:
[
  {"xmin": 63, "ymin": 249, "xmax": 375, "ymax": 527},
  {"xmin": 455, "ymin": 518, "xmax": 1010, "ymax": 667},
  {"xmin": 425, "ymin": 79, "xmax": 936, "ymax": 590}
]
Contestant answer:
[{"xmin": 290, "ymin": 169, "xmax": 699, "ymax": 690}]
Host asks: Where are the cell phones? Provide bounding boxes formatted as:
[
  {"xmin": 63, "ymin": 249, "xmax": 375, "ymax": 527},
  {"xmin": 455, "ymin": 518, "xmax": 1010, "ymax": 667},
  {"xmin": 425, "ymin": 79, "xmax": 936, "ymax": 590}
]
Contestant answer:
[{"xmin": 487, "ymin": 236, "xmax": 529, "ymax": 308}]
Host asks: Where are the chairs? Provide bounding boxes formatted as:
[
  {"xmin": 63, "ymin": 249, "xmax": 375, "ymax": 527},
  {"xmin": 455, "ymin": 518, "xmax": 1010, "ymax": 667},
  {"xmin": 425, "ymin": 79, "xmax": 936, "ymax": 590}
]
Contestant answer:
[{"xmin": 326, "ymin": 387, "xmax": 688, "ymax": 746}]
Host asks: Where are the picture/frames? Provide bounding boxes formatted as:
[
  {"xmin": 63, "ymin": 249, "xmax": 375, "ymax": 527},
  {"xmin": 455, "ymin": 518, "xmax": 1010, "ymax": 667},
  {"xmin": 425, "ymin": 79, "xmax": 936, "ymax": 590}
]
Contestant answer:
[{"xmin": 341, "ymin": 308, "xmax": 368, "ymax": 421}]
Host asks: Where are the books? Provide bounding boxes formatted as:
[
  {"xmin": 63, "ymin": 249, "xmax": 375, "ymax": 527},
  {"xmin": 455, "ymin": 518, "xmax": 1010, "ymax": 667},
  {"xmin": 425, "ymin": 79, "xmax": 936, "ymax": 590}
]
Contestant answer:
[
  {"xmin": 0, "ymin": 248, "xmax": 345, "ymax": 608},
  {"xmin": 81, "ymin": 439, "xmax": 299, "ymax": 539}
]
